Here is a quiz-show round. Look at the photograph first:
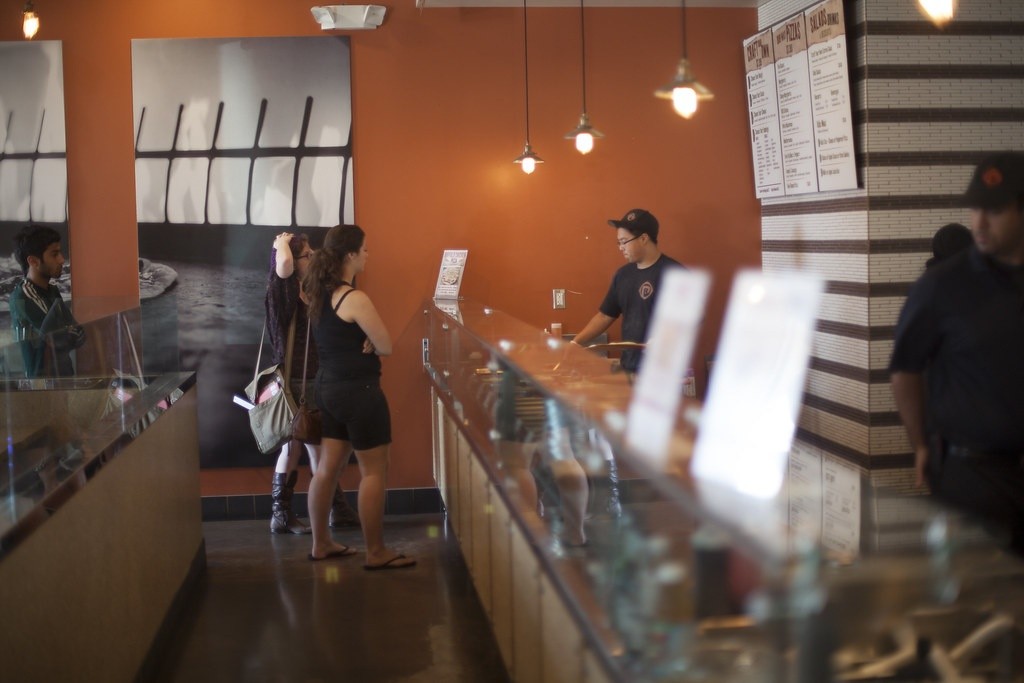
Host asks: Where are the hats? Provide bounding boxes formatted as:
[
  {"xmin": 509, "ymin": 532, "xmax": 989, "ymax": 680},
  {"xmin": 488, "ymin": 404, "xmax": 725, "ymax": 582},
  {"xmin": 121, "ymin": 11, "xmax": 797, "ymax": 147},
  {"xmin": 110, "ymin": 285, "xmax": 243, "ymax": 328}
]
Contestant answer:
[
  {"xmin": 608, "ymin": 208, "xmax": 659, "ymax": 238},
  {"xmin": 959, "ymin": 153, "xmax": 1024, "ymax": 209}
]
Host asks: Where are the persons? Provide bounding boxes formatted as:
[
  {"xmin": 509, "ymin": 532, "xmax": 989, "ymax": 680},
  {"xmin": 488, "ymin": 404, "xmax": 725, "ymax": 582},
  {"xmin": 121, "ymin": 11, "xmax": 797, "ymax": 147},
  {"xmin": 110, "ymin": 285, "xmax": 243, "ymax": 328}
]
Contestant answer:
[
  {"xmin": 561, "ymin": 210, "xmax": 686, "ymax": 387},
  {"xmin": 266, "ymin": 231, "xmax": 362, "ymax": 535},
  {"xmin": 308, "ymin": 224, "xmax": 418, "ymax": 570},
  {"xmin": 0, "ymin": 391, "xmax": 86, "ymax": 558},
  {"xmin": 887, "ymin": 152, "xmax": 1024, "ymax": 563},
  {"xmin": 491, "ymin": 351, "xmax": 622, "ymax": 548},
  {"xmin": 9, "ymin": 225, "xmax": 87, "ymax": 389}
]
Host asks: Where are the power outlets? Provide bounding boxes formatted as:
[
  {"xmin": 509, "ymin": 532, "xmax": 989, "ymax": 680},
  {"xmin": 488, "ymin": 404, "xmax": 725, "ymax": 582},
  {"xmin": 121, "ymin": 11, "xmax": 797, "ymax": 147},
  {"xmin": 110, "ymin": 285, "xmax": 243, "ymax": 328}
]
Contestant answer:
[{"xmin": 552, "ymin": 289, "xmax": 566, "ymax": 309}]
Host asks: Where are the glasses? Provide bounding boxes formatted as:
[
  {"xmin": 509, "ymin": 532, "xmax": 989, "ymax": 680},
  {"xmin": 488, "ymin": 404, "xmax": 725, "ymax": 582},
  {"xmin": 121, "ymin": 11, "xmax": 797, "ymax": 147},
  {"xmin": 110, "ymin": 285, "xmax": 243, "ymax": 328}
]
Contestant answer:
[
  {"xmin": 296, "ymin": 249, "xmax": 315, "ymax": 260},
  {"xmin": 617, "ymin": 235, "xmax": 641, "ymax": 245}
]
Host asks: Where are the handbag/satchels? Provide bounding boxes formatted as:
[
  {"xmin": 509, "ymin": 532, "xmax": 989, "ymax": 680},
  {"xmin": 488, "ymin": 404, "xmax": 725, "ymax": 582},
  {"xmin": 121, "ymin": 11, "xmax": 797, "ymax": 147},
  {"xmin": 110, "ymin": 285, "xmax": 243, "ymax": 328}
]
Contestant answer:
[
  {"xmin": 244, "ymin": 363, "xmax": 298, "ymax": 454},
  {"xmin": 291, "ymin": 403, "xmax": 324, "ymax": 446}
]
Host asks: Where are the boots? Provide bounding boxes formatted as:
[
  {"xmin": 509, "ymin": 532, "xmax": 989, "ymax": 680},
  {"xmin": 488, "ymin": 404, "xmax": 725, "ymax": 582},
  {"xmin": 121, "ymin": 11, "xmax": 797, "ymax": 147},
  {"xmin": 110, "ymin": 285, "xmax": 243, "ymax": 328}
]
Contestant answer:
[
  {"xmin": 270, "ymin": 470, "xmax": 312, "ymax": 535},
  {"xmin": 311, "ymin": 472, "xmax": 360, "ymax": 527}
]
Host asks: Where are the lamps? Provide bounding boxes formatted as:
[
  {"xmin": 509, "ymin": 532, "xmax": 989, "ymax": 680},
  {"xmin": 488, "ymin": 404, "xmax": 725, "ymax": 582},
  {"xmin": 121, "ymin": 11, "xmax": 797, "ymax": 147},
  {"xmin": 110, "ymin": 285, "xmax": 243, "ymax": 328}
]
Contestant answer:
[
  {"xmin": 564, "ymin": 0, "xmax": 603, "ymax": 154},
  {"xmin": 511, "ymin": 0, "xmax": 545, "ymax": 175},
  {"xmin": 654, "ymin": 0, "xmax": 713, "ymax": 117}
]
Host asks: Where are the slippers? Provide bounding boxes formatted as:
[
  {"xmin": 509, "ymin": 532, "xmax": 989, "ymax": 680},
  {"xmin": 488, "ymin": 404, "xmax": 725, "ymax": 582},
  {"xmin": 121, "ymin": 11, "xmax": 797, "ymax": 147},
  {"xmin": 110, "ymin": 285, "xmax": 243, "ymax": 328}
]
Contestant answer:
[
  {"xmin": 363, "ymin": 554, "xmax": 416, "ymax": 570},
  {"xmin": 307, "ymin": 544, "xmax": 357, "ymax": 561}
]
